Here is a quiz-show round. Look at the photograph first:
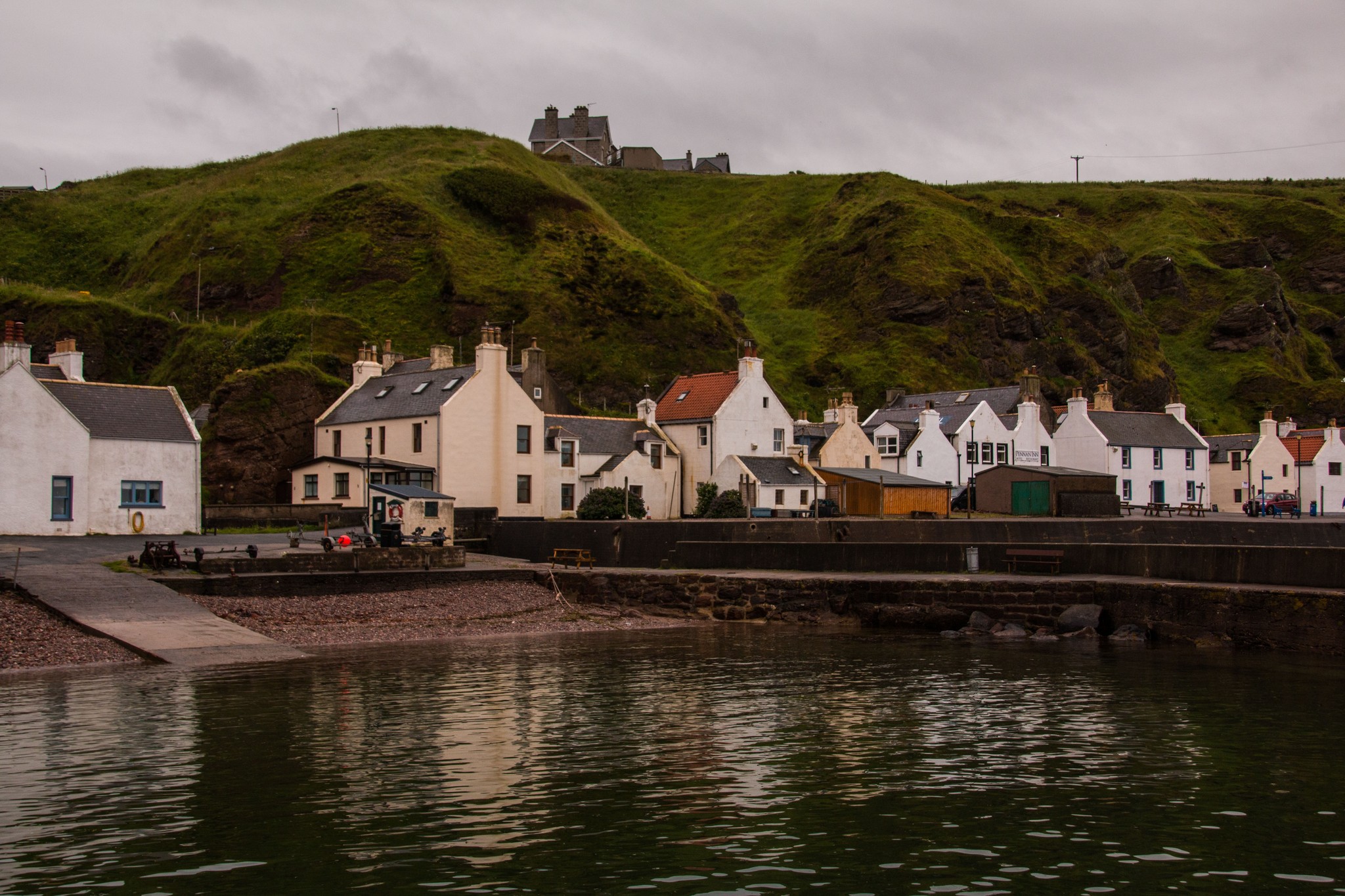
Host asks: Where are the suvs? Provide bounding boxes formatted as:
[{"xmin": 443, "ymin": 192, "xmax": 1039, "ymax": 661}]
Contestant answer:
[
  {"xmin": 1243, "ymin": 492, "xmax": 1297, "ymax": 516},
  {"xmin": 950, "ymin": 484, "xmax": 976, "ymax": 513}
]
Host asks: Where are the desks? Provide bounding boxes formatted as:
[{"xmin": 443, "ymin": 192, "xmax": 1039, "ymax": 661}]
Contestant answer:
[
  {"xmin": 551, "ymin": 549, "xmax": 593, "ymax": 570},
  {"xmin": 1177, "ymin": 502, "xmax": 1205, "ymax": 517},
  {"xmin": 789, "ymin": 510, "xmax": 815, "ymax": 518},
  {"xmin": 1144, "ymin": 503, "xmax": 1172, "ymax": 517},
  {"xmin": 1120, "ymin": 501, "xmax": 1131, "ymax": 515}
]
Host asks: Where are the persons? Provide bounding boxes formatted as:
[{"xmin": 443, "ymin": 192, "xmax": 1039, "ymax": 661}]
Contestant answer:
[{"xmin": 1342, "ymin": 497, "xmax": 1345, "ymax": 509}]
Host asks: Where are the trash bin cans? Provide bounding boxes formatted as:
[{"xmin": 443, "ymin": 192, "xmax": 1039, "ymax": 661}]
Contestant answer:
[
  {"xmin": 380, "ymin": 522, "xmax": 401, "ymax": 547},
  {"xmin": 1310, "ymin": 501, "xmax": 1316, "ymax": 516}
]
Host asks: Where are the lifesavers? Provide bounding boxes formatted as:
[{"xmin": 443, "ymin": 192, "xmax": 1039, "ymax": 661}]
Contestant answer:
[{"xmin": 389, "ymin": 504, "xmax": 402, "ymax": 519}]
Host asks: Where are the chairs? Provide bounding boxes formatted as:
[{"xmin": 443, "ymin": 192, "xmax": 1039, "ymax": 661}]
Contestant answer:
[
  {"xmin": 1286, "ymin": 495, "xmax": 1290, "ymax": 500},
  {"xmin": 1268, "ymin": 495, "xmax": 1272, "ymax": 500},
  {"xmin": 1278, "ymin": 496, "xmax": 1282, "ymax": 501},
  {"xmin": 1272, "ymin": 504, "xmax": 1282, "ymax": 519},
  {"xmin": 1291, "ymin": 504, "xmax": 1300, "ymax": 519}
]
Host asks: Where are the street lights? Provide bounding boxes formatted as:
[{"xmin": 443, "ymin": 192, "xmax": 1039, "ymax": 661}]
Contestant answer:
[
  {"xmin": 1296, "ymin": 433, "xmax": 1302, "ymax": 516},
  {"xmin": 970, "ymin": 419, "xmax": 976, "ymax": 511},
  {"xmin": 366, "ymin": 442, "xmax": 371, "ymax": 527}
]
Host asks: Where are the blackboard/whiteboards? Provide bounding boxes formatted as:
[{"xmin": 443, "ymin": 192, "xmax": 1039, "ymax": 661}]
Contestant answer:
[{"xmin": 1212, "ymin": 504, "xmax": 1218, "ymax": 512}]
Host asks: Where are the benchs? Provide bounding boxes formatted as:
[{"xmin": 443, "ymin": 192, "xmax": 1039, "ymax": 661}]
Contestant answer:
[
  {"xmin": 1120, "ymin": 506, "xmax": 1134, "ymax": 509},
  {"xmin": 1174, "ymin": 507, "xmax": 1193, "ymax": 511},
  {"xmin": 548, "ymin": 556, "xmax": 580, "ymax": 561},
  {"xmin": 1159, "ymin": 509, "xmax": 1175, "ymax": 511},
  {"xmin": 1191, "ymin": 509, "xmax": 1209, "ymax": 511},
  {"xmin": 1141, "ymin": 507, "xmax": 1163, "ymax": 511},
  {"xmin": 584, "ymin": 558, "xmax": 596, "ymax": 561}
]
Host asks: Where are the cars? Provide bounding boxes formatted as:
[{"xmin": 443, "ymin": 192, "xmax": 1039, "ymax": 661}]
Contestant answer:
[{"xmin": 810, "ymin": 499, "xmax": 840, "ymax": 517}]
